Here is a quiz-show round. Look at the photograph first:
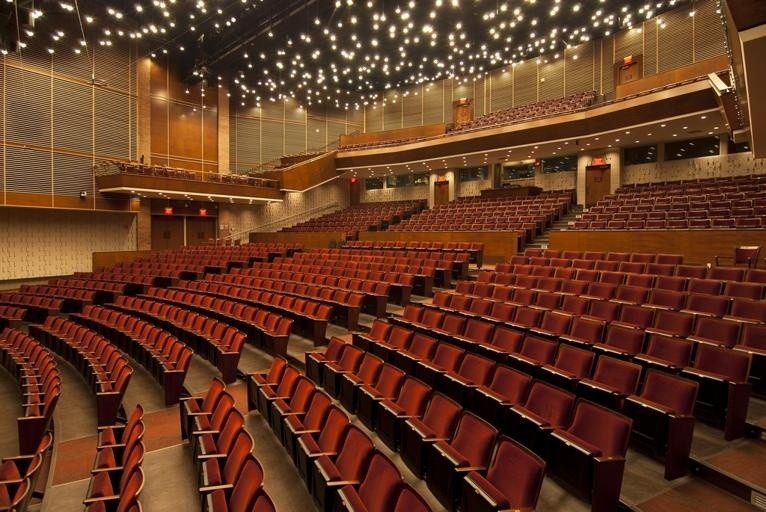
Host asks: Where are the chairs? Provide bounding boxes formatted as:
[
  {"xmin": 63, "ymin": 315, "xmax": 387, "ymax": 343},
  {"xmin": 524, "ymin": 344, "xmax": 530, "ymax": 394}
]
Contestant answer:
[
  {"xmin": 444, "ymin": 90, "xmax": 598, "ymax": 136},
  {"xmin": 0, "ymin": 174, "xmax": 766, "ymax": 512},
  {"xmin": 101, "ymin": 156, "xmax": 306, "ymax": 188},
  {"xmin": 338, "ymin": 135, "xmax": 426, "ymax": 152}
]
